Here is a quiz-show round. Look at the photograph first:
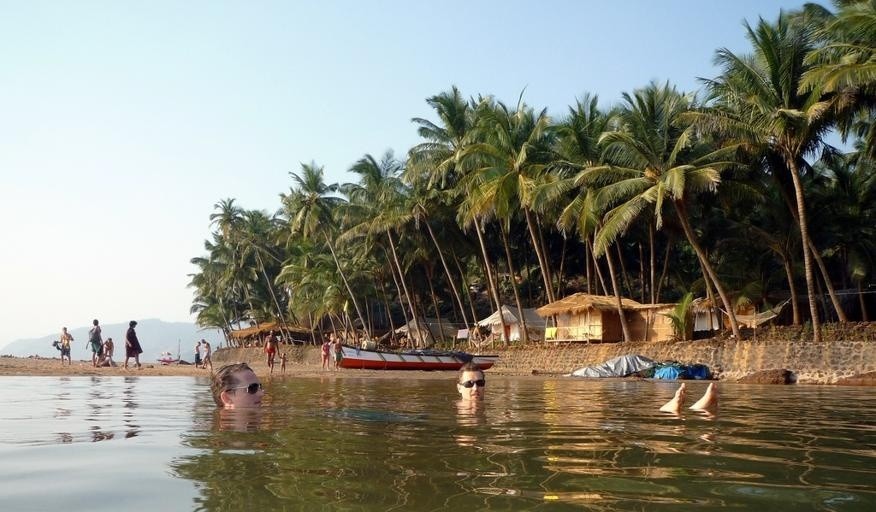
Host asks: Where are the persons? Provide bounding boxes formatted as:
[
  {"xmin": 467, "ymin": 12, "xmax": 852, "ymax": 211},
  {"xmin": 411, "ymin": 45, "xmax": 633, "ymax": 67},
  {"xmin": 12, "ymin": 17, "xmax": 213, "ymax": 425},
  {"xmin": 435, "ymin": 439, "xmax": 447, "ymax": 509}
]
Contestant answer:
[
  {"xmin": 263, "ymin": 330, "xmax": 280, "ymax": 374},
  {"xmin": 390, "ymin": 334, "xmax": 407, "ymax": 348},
  {"xmin": 255, "ymin": 337, "xmax": 259, "ymax": 346},
  {"xmin": 56, "ymin": 327, "xmax": 74, "ymax": 366},
  {"xmin": 87, "ymin": 319, "xmax": 115, "ymax": 367},
  {"xmin": 123, "ymin": 320, "xmax": 143, "ymax": 369},
  {"xmin": 278, "ymin": 353, "xmax": 289, "ymax": 374},
  {"xmin": 209, "ymin": 363, "xmax": 265, "ymax": 411},
  {"xmin": 453, "ymin": 363, "xmax": 718, "ymax": 413},
  {"xmin": 247, "ymin": 342, "xmax": 251, "ymax": 346},
  {"xmin": 321, "ymin": 337, "xmax": 345, "ymax": 371},
  {"xmin": 194, "ymin": 341, "xmax": 201, "ymax": 367},
  {"xmin": 202, "ymin": 338, "xmax": 213, "ymax": 370}
]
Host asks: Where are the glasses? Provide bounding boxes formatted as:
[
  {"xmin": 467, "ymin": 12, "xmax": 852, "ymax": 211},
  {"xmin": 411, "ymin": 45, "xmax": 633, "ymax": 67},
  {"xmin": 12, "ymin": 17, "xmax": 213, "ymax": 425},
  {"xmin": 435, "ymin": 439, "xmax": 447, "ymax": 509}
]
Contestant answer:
[
  {"xmin": 224, "ymin": 383, "xmax": 263, "ymax": 395},
  {"xmin": 458, "ymin": 379, "xmax": 486, "ymax": 388}
]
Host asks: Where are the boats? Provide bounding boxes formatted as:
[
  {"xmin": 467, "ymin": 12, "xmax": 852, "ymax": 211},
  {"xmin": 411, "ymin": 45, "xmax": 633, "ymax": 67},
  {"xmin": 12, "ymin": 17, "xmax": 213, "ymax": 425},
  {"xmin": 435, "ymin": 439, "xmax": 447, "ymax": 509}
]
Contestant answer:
[{"xmin": 326, "ymin": 341, "xmax": 499, "ymax": 373}]
